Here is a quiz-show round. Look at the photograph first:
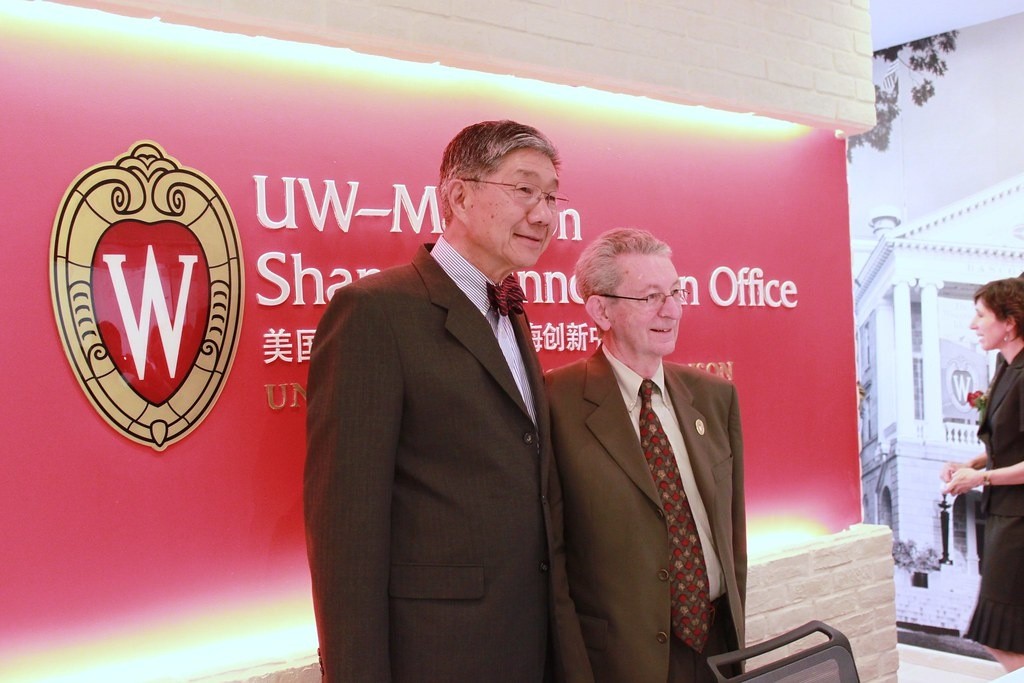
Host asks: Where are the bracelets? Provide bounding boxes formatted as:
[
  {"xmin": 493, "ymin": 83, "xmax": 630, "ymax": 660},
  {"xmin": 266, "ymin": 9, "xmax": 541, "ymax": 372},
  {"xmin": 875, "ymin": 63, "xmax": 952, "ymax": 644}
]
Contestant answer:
[{"xmin": 982, "ymin": 471, "xmax": 990, "ymax": 486}]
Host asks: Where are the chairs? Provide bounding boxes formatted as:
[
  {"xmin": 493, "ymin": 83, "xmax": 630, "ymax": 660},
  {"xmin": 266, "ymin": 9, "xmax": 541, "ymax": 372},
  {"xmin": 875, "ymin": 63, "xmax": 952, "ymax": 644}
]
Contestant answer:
[{"xmin": 706, "ymin": 619, "xmax": 861, "ymax": 683}]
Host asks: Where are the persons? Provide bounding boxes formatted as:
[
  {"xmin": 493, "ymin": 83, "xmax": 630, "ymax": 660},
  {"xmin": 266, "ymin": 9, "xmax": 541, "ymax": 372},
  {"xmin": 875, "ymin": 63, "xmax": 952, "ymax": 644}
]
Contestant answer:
[
  {"xmin": 545, "ymin": 228, "xmax": 748, "ymax": 683},
  {"xmin": 303, "ymin": 121, "xmax": 559, "ymax": 683},
  {"xmin": 940, "ymin": 278, "xmax": 1024, "ymax": 672}
]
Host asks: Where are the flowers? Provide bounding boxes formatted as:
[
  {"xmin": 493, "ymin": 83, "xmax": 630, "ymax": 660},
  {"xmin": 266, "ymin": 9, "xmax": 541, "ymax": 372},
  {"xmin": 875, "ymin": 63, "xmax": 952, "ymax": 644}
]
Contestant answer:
[{"xmin": 966, "ymin": 390, "xmax": 987, "ymax": 424}]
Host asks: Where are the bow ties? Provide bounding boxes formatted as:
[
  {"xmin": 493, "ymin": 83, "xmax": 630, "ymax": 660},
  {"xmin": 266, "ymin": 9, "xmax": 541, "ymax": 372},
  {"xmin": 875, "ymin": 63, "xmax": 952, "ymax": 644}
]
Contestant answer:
[{"xmin": 486, "ymin": 274, "xmax": 527, "ymax": 317}]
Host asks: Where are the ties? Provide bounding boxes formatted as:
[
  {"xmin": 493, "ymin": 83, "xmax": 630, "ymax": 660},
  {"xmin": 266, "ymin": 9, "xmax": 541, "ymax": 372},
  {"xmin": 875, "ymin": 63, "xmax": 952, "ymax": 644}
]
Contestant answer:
[{"xmin": 637, "ymin": 379, "xmax": 711, "ymax": 654}]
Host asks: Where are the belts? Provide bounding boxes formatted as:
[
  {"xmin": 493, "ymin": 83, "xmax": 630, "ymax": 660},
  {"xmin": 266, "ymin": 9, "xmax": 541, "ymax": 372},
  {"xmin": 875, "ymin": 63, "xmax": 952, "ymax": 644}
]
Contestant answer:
[{"xmin": 709, "ymin": 595, "xmax": 728, "ymax": 627}]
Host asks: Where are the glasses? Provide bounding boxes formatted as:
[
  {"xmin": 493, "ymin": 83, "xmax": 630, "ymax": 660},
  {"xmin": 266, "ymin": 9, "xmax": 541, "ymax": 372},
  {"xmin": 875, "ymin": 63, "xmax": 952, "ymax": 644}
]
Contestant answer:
[
  {"xmin": 597, "ymin": 289, "xmax": 690, "ymax": 308},
  {"xmin": 462, "ymin": 179, "xmax": 569, "ymax": 213}
]
